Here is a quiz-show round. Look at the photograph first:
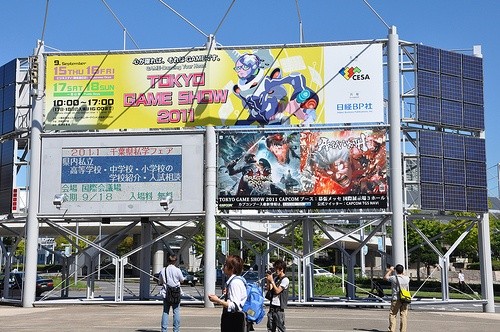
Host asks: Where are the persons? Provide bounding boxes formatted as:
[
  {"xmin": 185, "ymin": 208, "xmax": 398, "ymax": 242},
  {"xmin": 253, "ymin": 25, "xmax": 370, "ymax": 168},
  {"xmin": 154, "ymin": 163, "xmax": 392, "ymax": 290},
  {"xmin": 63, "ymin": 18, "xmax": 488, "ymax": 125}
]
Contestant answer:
[
  {"xmin": 458, "ymin": 270, "xmax": 466, "ymax": 294},
  {"xmin": 265, "ymin": 260, "xmax": 289, "ymax": 332},
  {"xmin": 383, "ymin": 264, "xmax": 410, "ymax": 332},
  {"xmin": 159, "ymin": 254, "xmax": 184, "ymax": 332},
  {"xmin": 208, "ymin": 255, "xmax": 249, "ymax": 332}
]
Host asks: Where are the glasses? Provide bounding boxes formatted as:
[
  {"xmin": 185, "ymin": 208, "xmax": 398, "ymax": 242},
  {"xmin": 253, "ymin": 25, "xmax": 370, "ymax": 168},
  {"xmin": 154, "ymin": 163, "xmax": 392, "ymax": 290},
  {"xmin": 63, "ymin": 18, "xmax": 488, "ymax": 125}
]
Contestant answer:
[{"xmin": 223, "ymin": 261, "xmax": 226, "ymax": 265}]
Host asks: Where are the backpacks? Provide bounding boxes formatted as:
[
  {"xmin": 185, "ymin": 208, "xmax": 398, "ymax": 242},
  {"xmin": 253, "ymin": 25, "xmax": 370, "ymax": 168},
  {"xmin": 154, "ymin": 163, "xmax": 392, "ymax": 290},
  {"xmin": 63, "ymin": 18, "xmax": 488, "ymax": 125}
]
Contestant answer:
[
  {"xmin": 242, "ymin": 282, "xmax": 266, "ymax": 324},
  {"xmin": 399, "ymin": 290, "xmax": 411, "ymax": 304}
]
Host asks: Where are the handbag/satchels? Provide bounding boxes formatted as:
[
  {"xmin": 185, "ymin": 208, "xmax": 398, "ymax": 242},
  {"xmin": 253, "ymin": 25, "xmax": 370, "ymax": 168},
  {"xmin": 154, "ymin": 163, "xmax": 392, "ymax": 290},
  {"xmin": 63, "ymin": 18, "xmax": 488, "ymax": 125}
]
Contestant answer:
[
  {"xmin": 166, "ymin": 287, "xmax": 180, "ymax": 301},
  {"xmin": 221, "ymin": 302, "xmax": 247, "ymax": 332},
  {"xmin": 265, "ymin": 288, "xmax": 274, "ymax": 300}
]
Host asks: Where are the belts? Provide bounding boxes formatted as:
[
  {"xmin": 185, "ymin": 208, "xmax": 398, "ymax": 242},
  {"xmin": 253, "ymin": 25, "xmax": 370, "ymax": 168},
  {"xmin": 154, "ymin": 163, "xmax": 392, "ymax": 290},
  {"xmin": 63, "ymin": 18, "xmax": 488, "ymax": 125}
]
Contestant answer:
[{"xmin": 270, "ymin": 308, "xmax": 284, "ymax": 312}]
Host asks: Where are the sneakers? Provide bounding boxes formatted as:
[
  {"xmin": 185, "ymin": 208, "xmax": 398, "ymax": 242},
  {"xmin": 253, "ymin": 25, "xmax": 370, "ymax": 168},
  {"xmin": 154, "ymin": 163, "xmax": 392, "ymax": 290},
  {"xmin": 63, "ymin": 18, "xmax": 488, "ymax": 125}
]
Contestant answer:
[{"xmin": 387, "ymin": 327, "xmax": 390, "ymax": 331}]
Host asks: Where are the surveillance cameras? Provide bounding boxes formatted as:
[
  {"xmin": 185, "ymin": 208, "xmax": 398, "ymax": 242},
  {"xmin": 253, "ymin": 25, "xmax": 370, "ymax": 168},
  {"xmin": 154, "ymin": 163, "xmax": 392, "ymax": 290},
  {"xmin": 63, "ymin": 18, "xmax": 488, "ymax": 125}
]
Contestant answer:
[
  {"xmin": 54, "ymin": 194, "xmax": 64, "ymax": 205},
  {"xmin": 160, "ymin": 197, "xmax": 172, "ymax": 207}
]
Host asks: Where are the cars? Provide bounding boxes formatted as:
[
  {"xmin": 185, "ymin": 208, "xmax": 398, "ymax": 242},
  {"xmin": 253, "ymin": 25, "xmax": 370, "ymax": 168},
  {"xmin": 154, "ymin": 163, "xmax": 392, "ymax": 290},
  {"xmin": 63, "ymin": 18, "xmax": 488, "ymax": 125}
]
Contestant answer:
[
  {"xmin": 0, "ymin": 272, "xmax": 55, "ymax": 297},
  {"xmin": 193, "ymin": 264, "xmax": 281, "ymax": 290},
  {"xmin": 153, "ymin": 267, "xmax": 200, "ymax": 287},
  {"xmin": 301, "ymin": 268, "xmax": 333, "ymax": 278}
]
